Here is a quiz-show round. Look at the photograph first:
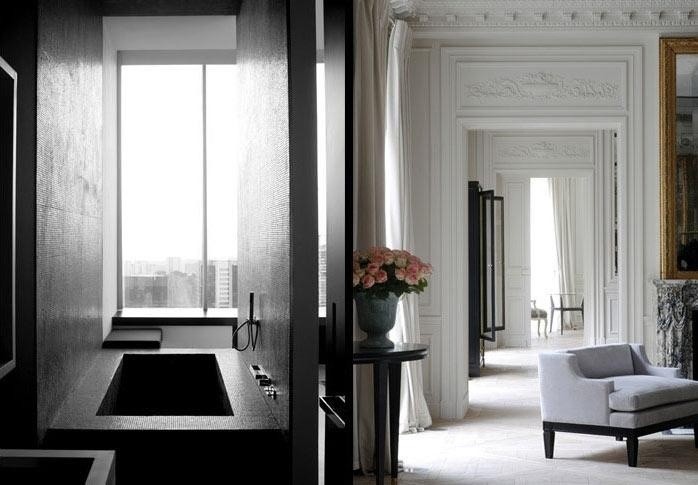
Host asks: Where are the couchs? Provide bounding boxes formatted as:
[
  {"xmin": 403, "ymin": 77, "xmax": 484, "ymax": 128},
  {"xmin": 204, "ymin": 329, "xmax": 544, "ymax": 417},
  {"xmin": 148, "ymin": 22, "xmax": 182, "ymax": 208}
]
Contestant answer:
[{"xmin": 539, "ymin": 342, "xmax": 697, "ymax": 467}]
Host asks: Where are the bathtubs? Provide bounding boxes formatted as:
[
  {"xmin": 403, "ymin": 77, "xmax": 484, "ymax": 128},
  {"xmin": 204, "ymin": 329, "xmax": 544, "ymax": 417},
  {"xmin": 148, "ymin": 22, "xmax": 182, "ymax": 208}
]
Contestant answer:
[{"xmin": 97, "ymin": 354, "xmax": 235, "ymax": 416}]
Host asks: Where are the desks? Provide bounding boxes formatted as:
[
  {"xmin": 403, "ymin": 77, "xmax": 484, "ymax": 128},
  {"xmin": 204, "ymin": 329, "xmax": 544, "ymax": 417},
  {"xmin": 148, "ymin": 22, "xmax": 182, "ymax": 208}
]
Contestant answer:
[
  {"xmin": 348, "ymin": 340, "xmax": 431, "ymax": 484},
  {"xmin": 549, "ymin": 292, "xmax": 585, "ymax": 335}
]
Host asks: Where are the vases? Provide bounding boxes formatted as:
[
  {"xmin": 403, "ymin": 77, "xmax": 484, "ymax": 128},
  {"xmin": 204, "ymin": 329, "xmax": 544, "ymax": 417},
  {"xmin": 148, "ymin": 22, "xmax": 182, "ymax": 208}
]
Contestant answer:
[{"xmin": 356, "ymin": 289, "xmax": 398, "ymax": 350}]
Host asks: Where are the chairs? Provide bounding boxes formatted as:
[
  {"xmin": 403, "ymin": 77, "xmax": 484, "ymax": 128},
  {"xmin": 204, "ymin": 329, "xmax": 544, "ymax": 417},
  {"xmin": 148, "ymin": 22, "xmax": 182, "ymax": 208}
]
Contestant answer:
[{"xmin": 531, "ymin": 300, "xmax": 548, "ymax": 337}]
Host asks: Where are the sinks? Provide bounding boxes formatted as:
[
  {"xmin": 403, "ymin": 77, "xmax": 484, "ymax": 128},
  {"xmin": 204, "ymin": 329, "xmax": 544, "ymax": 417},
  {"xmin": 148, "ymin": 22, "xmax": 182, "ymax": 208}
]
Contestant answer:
[{"xmin": 1, "ymin": 450, "xmax": 93, "ymax": 484}]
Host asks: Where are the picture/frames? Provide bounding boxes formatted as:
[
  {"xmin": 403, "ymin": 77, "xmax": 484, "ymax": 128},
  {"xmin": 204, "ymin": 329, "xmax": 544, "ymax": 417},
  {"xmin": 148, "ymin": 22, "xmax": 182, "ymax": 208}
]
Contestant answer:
[{"xmin": 658, "ymin": 31, "xmax": 697, "ymax": 278}]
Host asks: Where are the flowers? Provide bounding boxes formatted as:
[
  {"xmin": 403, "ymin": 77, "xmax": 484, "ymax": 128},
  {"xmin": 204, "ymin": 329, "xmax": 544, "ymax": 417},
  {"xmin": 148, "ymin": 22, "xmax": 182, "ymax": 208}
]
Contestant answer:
[{"xmin": 353, "ymin": 246, "xmax": 433, "ymax": 301}]
